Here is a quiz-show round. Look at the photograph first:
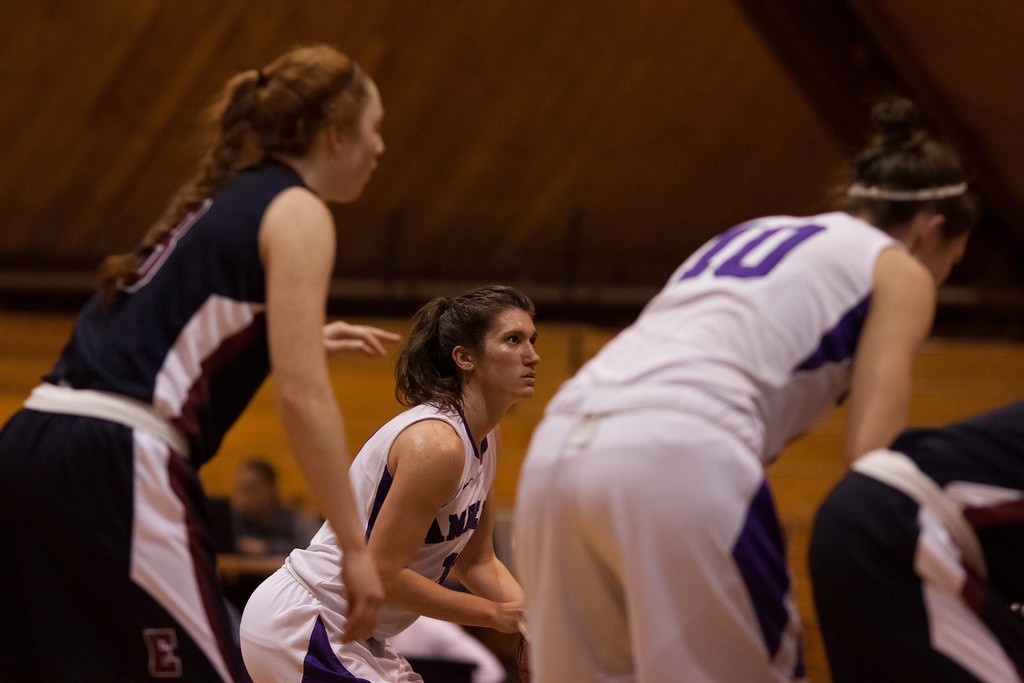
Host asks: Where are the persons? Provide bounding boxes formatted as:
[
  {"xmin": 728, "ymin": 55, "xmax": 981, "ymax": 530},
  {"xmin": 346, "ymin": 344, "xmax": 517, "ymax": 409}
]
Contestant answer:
[
  {"xmin": 0, "ymin": 43, "xmax": 402, "ymax": 681},
  {"xmin": 230, "ymin": 457, "xmax": 313, "ymax": 553},
  {"xmin": 807, "ymin": 397, "xmax": 1023, "ymax": 682},
  {"xmin": 239, "ymin": 279, "xmax": 543, "ymax": 680},
  {"xmin": 511, "ymin": 87, "xmax": 981, "ymax": 680}
]
